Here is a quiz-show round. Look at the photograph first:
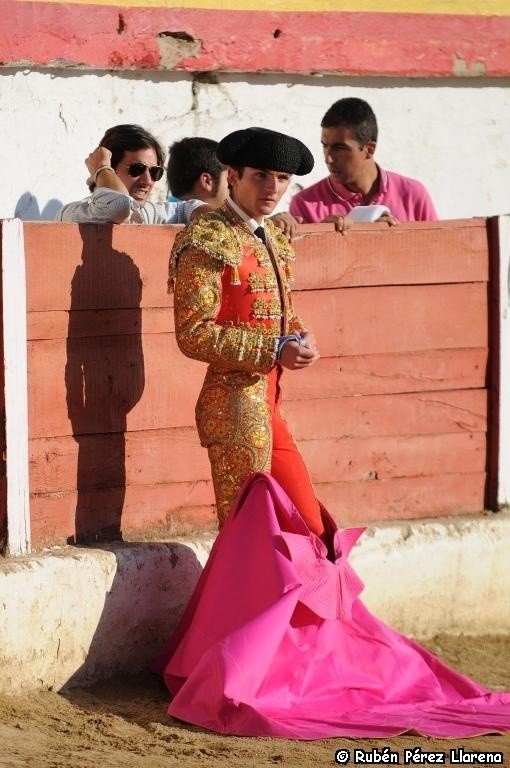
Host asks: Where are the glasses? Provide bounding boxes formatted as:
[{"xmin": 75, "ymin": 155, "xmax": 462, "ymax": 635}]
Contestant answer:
[{"xmin": 118, "ymin": 162, "xmax": 164, "ymax": 182}]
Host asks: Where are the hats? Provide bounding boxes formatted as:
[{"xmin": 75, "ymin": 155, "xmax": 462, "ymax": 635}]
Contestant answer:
[{"xmin": 214, "ymin": 127, "xmax": 314, "ymax": 176}]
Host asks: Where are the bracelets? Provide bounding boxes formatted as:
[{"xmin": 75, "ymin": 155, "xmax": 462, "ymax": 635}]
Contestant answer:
[{"xmin": 94, "ymin": 165, "xmax": 115, "ymax": 183}]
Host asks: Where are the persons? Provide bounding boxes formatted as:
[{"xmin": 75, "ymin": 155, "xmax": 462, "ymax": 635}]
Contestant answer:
[
  {"xmin": 167, "ymin": 125, "xmax": 331, "ymax": 632},
  {"xmin": 167, "ymin": 136, "xmax": 352, "ymax": 235},
  {"xmin": 55, "ymin": 123, "xmax": 216, "ymax": 225},
  {"xmin": 290, "ymin": 96, "xmax": 438, "ymax": 227}
]
